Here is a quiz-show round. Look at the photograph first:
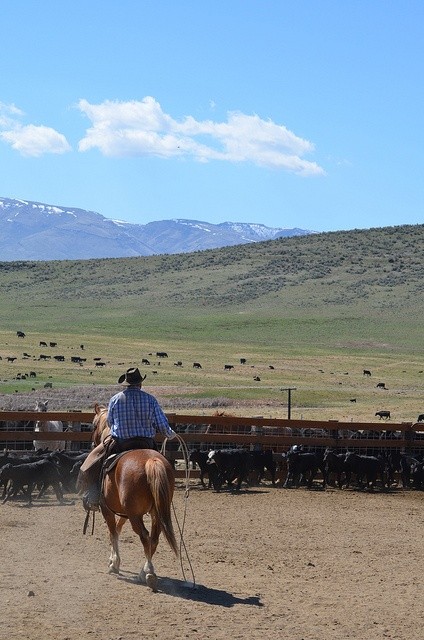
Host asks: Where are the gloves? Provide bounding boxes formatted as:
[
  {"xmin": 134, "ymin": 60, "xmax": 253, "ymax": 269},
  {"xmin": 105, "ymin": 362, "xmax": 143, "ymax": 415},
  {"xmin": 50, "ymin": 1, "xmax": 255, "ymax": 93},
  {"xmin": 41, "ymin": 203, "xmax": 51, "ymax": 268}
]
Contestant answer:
[{"xmin": 167, "ymin": 430, "xmax": 177, "ymax": 441}]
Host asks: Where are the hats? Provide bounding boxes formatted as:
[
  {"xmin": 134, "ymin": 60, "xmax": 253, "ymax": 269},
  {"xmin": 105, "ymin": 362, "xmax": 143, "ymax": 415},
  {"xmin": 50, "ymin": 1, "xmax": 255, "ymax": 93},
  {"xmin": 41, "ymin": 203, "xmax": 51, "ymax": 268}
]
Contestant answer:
[{"xmin": 118, "ymin": 367, "xmax": 146, "ymax": 386}]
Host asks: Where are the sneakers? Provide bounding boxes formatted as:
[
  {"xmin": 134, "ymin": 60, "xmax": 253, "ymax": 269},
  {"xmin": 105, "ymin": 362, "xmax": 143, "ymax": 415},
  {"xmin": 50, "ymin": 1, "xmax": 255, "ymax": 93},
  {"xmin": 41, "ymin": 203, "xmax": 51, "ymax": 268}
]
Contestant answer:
[{"xmin": 83, "ymin": 496, "xmax": 100, "ymax": 510}]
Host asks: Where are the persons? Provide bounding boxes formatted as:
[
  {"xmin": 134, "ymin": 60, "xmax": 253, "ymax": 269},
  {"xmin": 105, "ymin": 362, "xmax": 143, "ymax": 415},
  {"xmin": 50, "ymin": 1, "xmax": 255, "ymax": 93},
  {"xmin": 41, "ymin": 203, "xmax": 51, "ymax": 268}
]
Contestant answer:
[{"xmin": 82, "ymin": 367, "xmax": 177, "ymax": 512}]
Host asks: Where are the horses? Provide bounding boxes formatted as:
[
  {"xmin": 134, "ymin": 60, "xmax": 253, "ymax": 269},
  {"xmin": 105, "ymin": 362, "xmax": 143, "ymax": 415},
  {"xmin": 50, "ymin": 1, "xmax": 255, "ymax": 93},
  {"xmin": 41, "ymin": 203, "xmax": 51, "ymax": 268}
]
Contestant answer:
[
  {"xmin": 91, "ymin": 402, "xmax": 180, "ymax": 591},
  {"xmin": 33, "ymin": 399, "xmax": 67, "ymax": 452},
  {"xmin": 205, "ymin": 410, "xmax": 252, "ymax": 434}
]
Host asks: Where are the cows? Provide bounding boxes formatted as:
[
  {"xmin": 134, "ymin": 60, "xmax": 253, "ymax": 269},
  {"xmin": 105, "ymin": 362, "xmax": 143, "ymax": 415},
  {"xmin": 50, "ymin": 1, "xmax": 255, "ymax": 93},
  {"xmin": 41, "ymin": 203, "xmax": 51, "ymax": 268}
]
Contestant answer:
[
  {"xmin": 343, "ymin": 448, "xmax": 382, "ymax": 492},
  {"xmin": 375, "ymin": 410, "xmax": 391, "ymax": 420},
  {"xmin": 322, "ymin": 447, "xmax": 362, "ymax": 491},
  {"xmin": 384, "ymin": 449, "xmax": 424, "ymax": 491},
  {"xmin": 1, "ymin": 441, "xmax": 93, "ymax": 508},
  {"xmin": 188, "ymin": 446, "xmax": 246, "ymax": 493},
  {"xmin": 206, "ymin": 447, "xmax": 277, "ymax": 494},
  {"xmin": 283, "ymin": 449, "xmax": 329, "ymax": 490}
]
